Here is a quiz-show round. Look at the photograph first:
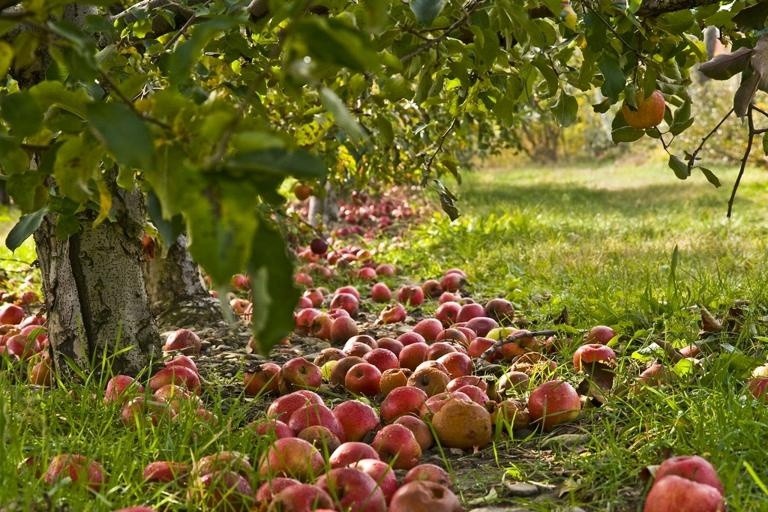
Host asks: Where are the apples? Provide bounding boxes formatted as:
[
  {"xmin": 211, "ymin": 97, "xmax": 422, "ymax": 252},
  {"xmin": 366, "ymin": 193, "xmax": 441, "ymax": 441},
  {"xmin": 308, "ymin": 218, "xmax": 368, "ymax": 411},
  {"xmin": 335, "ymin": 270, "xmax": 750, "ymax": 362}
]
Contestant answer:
[
  {"xmin": 107, "ymin": 182, "xmax": 768, "ymax": 512},
  {"xmin": 45, "ymin": 454, "xmax": 102, "ymax": 499},
  {"xmin": 0, "ymin": 291, "xmax": 53, "ymax": 387},
  {"xmin": 622, "ymin": 89, "xmax": 665, "ymax": 129}
]
